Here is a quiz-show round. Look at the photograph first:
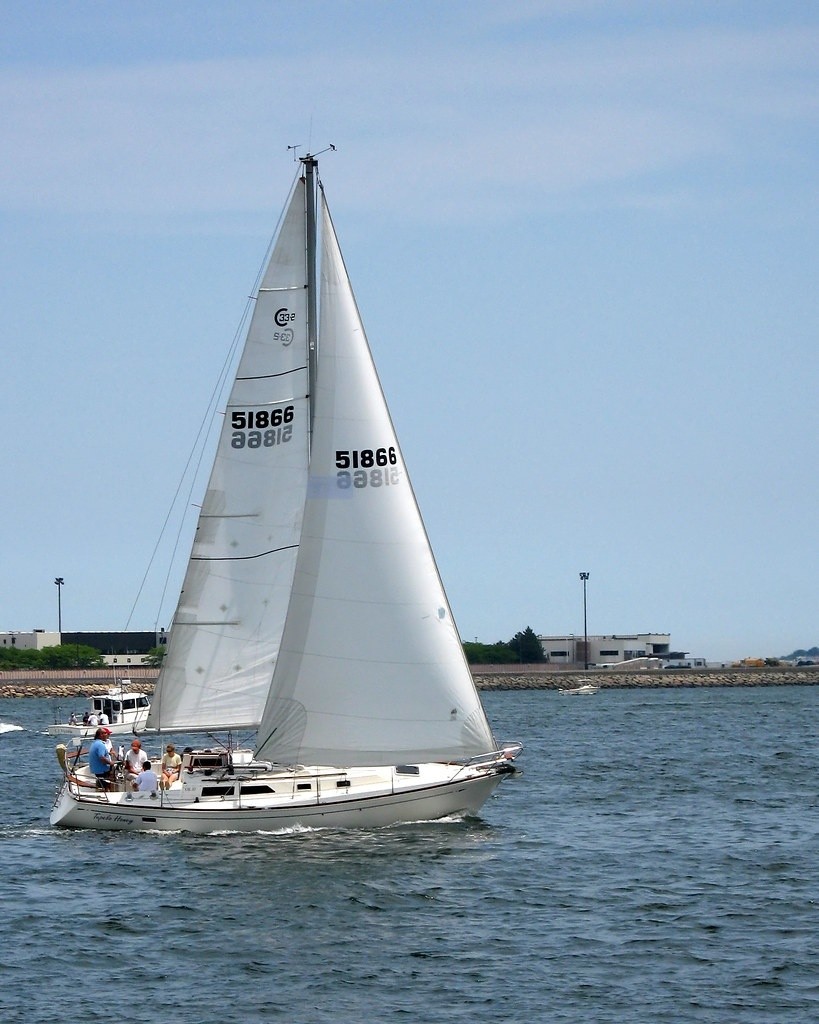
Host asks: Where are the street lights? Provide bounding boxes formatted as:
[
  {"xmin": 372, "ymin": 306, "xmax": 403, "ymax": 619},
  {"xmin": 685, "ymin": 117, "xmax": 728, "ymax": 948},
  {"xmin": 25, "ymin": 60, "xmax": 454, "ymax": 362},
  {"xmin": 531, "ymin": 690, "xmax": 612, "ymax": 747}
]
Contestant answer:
[
  {"xmin": 579, "ymin": 572, "xmax": 590, "ymax": 670},
  {"xmin": 53, "ymin": 577, "xmax": 64, "ymax": 632}
]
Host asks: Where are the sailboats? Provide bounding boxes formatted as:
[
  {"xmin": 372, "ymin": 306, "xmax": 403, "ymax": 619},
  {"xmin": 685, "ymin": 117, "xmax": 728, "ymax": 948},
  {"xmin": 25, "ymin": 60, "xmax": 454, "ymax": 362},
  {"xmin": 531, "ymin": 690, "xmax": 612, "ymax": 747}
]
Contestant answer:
[{"xmin": 48, "ymin": 142, "xmax": 526, "ymax": 834}]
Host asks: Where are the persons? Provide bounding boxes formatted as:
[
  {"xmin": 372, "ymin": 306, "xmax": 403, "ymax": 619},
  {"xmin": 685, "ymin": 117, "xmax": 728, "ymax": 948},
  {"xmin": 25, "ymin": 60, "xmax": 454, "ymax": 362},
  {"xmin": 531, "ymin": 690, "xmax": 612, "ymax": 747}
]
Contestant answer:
[
  {"xmin": 67, "ymin": 711, "xmax": 158, "ymax": 792},
  {"xmin": 159, "ymin": 744, "xmax": 182, "ymax": 790}
]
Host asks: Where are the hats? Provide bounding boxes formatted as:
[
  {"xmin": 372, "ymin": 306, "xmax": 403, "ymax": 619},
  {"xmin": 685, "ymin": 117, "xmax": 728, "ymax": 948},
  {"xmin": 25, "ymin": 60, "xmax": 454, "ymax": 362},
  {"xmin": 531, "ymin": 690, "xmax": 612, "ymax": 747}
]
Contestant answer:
[{"xmin": 103, "ymin": 728, "xmax": 112, "ymax": 734}]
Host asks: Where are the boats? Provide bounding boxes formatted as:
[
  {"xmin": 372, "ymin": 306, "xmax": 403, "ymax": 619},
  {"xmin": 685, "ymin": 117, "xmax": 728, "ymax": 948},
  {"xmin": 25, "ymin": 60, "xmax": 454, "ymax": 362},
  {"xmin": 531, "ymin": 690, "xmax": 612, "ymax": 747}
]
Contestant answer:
[
  {"xmin": 48, "ymin": 645, "xmax": 152, "ymax": 740},
  {"xmin": 558, "ymin": 678, "xmax": 601, "ymax": 695},
  {"xmin": 731, "ymin": 655, "xmax": 819, "ymax": 668}
]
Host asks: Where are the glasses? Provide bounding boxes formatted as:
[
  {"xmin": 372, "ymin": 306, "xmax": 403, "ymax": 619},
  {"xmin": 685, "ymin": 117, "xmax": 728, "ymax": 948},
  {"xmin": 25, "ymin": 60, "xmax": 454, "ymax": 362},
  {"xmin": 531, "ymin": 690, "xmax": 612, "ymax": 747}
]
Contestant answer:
[
  {"xmin": 108, "ymin": 733, "xmax": 110, "ymax": 735},
  {"xmin": 102, "ymin": 732, "xmax": 106, "ymax": 734},
  {"xmin": 166, "ymin": 750, "xmax": 173, "ymax": 753}
]
